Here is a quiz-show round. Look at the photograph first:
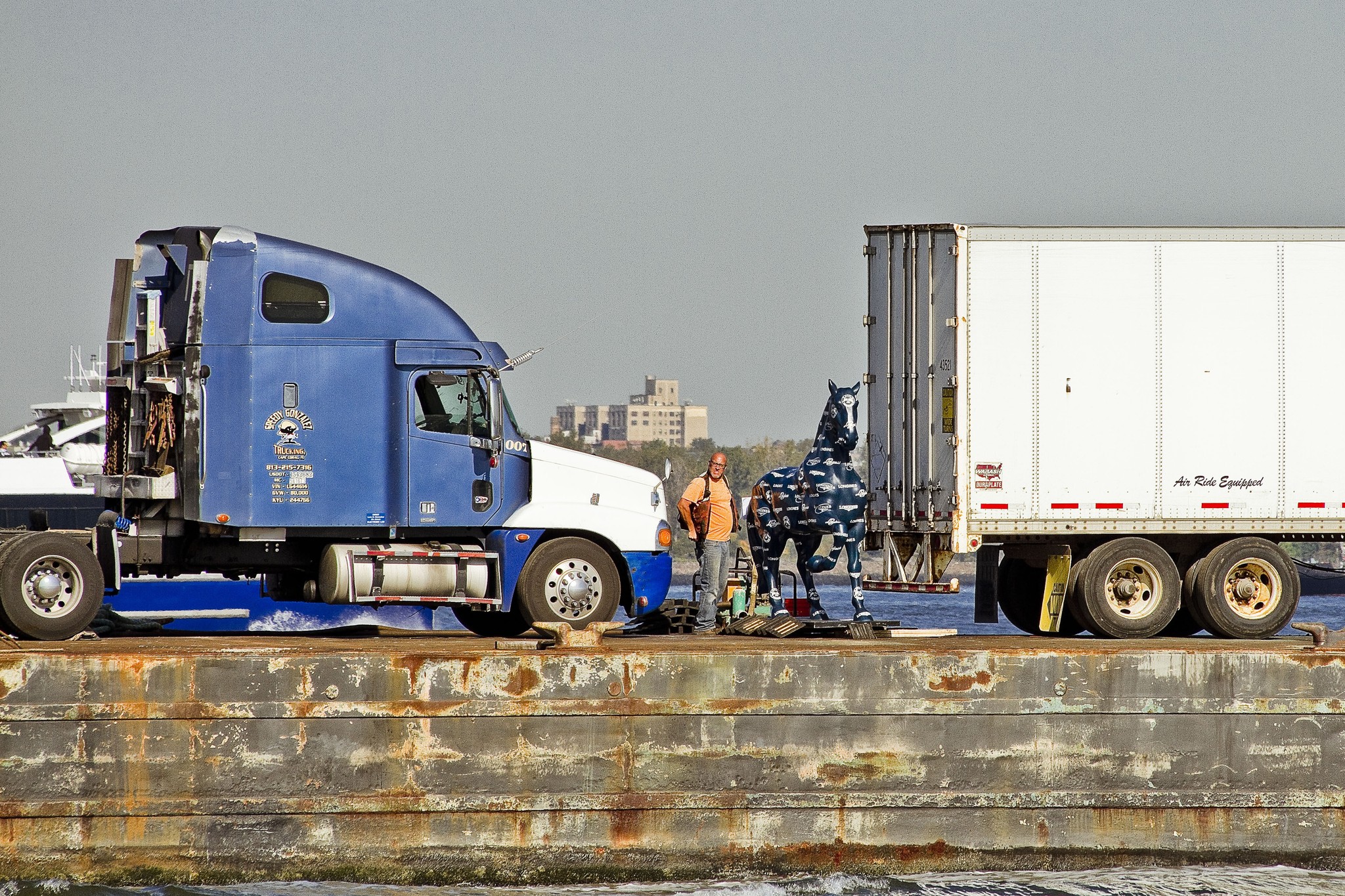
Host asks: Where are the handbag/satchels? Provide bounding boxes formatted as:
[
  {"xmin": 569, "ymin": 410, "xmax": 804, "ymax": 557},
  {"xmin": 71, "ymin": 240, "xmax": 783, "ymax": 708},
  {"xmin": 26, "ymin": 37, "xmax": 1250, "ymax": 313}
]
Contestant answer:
[{"xmin": 680, "ymin": 501, "xmax": 711, "ymax": 535}]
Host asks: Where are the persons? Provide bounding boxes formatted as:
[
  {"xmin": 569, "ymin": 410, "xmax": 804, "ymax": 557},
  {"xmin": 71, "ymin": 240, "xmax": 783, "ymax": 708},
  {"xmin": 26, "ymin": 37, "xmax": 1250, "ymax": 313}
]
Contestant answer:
[
  {"xmin": 28, "ymin": 424, "xmax": 56, "ymax": 456},
  {"xmin": 0, "ymin": 441, "xmax": 10, "ymax": 456},
  {"xmin": 677, "ymin": 452, "xmax": 741, "ymax": 631},
  {"xmin": 58, "ymin": 420, "xmax": 99, "ymax": 450}
]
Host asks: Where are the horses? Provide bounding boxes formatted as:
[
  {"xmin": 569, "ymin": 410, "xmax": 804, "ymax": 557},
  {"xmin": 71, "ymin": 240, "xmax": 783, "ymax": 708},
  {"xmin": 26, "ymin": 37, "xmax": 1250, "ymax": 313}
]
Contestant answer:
[{"xmin": 744, "ymin": 378, "xmax": 875, "ymax": 624}]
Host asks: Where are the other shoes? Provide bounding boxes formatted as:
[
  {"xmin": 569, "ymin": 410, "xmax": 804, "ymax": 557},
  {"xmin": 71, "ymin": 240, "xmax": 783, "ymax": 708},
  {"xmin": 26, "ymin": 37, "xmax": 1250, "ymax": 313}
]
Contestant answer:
[{"xmin": 693, "ymin": 629, "xmax": 716, "ymax": 635}]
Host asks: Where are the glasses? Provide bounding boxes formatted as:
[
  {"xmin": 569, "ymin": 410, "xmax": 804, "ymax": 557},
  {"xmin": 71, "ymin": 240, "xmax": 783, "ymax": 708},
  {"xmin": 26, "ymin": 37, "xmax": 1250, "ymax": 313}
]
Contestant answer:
[{"xmin": 710, "ymin": 461, "xmax": 727, "ymax": 469}]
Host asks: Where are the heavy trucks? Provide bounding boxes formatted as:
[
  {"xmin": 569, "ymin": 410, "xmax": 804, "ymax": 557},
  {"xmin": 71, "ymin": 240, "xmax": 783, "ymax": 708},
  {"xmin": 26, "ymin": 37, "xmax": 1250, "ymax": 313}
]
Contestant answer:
[
  {"xmin": 856, "ymin": 225, "xmax": 1345, "ymax": 639},
  {"xmin": 1, "ymin": 221, "xmax": 676, "ymax": 641}
]
